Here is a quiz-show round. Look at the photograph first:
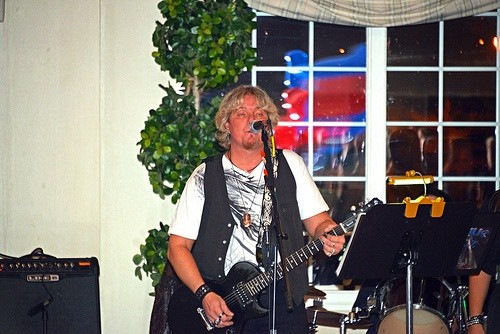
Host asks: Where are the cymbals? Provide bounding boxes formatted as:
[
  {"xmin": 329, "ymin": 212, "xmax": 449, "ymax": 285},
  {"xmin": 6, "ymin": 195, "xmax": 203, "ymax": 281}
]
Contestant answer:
[{"xmin": 304, "ymin": 289, "xmax": 326, "ymax": 297}]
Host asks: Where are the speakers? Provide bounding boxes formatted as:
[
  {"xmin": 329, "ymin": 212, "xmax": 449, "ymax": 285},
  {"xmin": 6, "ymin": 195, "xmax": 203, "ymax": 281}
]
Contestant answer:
[{"xmin": 0, "ymin": 257, "xmax": 101, "ymax": 334}]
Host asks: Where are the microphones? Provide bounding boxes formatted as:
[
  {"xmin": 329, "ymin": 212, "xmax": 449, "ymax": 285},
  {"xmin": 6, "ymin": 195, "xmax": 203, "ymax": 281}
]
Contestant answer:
[
  {"xmin": 28, "ymin": 299, "xmax": 52, "ymax": 318},
  {"xmin": 249, "ymin": 120, "xmax": 264, "ymax": 134}
]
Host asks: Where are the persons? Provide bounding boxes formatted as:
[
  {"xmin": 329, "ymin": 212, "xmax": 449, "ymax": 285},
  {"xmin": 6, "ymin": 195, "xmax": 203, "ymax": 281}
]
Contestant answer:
[
  {"xmin": 467, "ymin": 190, "xmax": 500, "ymax": 334},
  {"xmin": 167, "ymin": 84, "xmax": 346, "ymax": 334},
  {"xmin": 311, "ymin": 130, "xmax": 485, "ymax": 228}
]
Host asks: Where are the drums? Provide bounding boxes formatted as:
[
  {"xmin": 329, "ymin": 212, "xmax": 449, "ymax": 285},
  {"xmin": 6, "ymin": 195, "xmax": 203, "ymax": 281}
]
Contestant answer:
[{"xmin": 370, "ymin": 274, "xmax": 460, "ymax": 334}]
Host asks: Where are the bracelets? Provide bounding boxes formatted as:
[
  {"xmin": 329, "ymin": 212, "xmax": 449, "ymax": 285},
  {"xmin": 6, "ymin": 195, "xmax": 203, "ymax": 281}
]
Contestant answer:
[
  {"xmin": 467, "ymin": 314, "xmax": 484, "ymax": 326},
  {"xmin": 195, "ymin": 284, "xmax": 212, "ymax": 302}
]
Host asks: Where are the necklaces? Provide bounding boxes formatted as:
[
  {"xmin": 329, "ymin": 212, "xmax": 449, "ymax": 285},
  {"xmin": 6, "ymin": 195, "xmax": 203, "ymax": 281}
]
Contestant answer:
[{"xmin": 228, "ymin": 149, "xmax": 265, "ymax": 226}]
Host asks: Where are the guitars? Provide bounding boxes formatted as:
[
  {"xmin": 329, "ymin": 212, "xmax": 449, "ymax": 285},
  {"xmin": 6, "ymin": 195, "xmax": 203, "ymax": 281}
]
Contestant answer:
[{"xmin": 167, "ymin": 197, "xmax": 383, "ymax": 334}]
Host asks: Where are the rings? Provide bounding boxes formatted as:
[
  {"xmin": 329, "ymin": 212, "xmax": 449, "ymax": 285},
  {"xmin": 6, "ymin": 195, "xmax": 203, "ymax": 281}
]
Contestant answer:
[
  {"xmin": 210, "ymin": 313, "xmax": 225, "ymax": 327},
  {"xmin": 327, "ymin": 243, "xmax": 335, "ymax": 257}
]
